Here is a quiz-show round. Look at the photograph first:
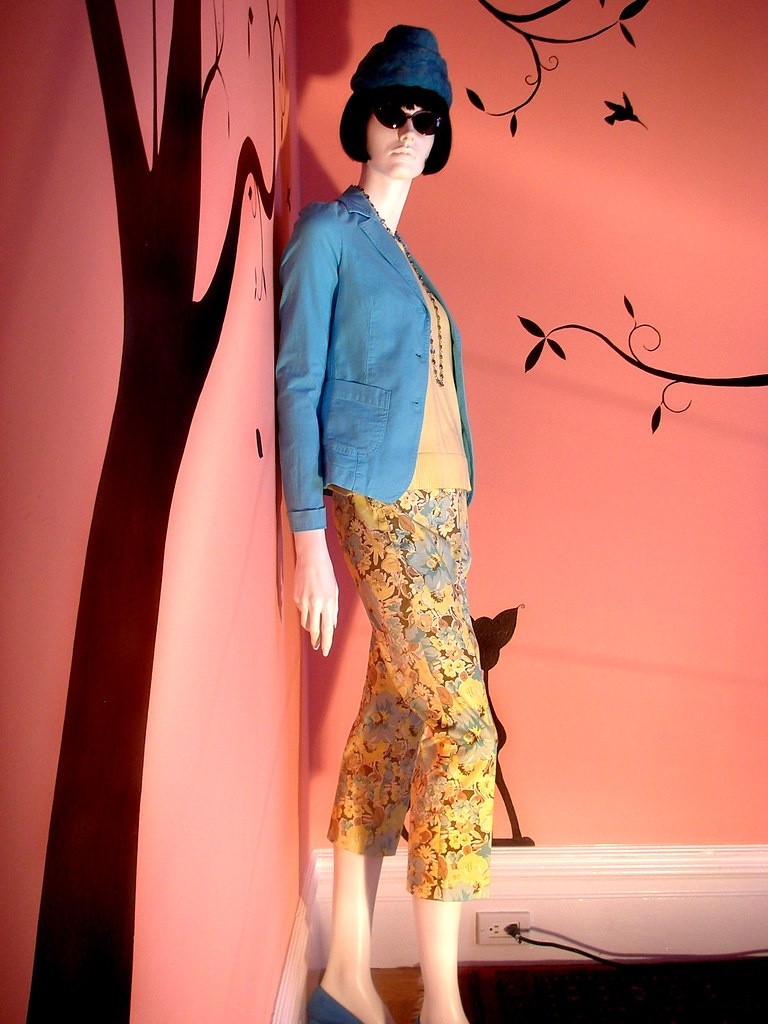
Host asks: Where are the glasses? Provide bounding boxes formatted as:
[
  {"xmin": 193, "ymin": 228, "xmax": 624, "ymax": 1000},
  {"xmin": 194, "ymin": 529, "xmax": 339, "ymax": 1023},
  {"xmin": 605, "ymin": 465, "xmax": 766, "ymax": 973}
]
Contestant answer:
[{"xmin": 370, "ymin": 103, "xmax": 442, "ymax": 137}]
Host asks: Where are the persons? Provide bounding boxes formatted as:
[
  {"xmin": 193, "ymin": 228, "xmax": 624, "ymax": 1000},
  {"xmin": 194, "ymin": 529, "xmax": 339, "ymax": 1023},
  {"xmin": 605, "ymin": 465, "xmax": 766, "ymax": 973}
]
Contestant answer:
[{"xmin": 276, "ymin": 22, "xmax": 500, "ymax": 1024}]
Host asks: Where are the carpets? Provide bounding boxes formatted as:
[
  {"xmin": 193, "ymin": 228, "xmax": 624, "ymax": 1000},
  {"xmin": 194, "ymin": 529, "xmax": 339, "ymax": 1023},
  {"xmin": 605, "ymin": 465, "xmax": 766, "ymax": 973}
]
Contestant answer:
[{"xmin": 411, "ymin": 957, "xmax": 768, "ymax": 1024}]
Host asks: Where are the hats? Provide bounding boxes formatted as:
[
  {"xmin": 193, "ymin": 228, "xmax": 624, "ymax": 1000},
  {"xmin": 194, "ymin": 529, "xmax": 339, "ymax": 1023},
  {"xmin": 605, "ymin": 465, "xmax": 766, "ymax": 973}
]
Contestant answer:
[{"xmin": 348, "ymin": 24, "xmax": 453, "ymax": 108}]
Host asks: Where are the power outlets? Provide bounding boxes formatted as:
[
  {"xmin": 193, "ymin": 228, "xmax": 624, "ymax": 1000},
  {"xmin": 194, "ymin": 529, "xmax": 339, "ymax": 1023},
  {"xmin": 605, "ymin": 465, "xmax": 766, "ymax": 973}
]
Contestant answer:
[{"xmin": 476, "ymin": 911, "xmax": 531, "ymax": 945}]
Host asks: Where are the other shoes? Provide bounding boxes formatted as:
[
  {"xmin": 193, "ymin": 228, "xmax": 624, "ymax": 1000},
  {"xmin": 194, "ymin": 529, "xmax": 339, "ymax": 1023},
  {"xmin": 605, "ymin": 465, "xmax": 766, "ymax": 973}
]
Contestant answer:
[{"xmin": 305, "ymin": 986, "xmax": 363, "ymax": 1024}]
